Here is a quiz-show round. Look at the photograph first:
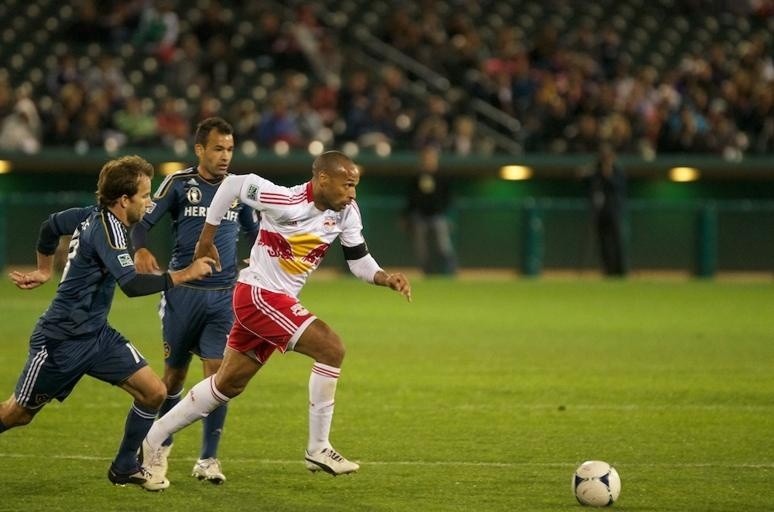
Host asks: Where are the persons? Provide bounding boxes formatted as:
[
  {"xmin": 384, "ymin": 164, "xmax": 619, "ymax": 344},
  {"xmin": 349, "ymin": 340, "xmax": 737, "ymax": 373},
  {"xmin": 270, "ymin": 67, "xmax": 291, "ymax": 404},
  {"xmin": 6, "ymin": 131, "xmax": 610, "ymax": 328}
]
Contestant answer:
[
  {"xmin": 1, "ymin": 2, "xmax": 774, "ymax": 155},
  {"xmin": 406, "ymin": 144, "xmax": 455, "ymax": 277},
  {"xmin": 1, "ymin": 155, "xmax": 216, "ymax": 490},
  {"xmin": 129, "ymin": 117, "xmax": 260, "ymax": 485},
  {"xmin": 134, "ymin": 151, "xmax": 412, "ymax": 491},
  {"xmin": 588, "ymin": 147, "xmax": 629, "ymax": 276}
]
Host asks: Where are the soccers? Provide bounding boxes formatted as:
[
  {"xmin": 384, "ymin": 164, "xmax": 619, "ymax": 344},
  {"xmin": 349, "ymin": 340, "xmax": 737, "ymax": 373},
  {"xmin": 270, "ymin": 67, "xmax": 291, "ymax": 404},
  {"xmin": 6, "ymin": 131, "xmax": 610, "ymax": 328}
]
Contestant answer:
[{"xmin": 572, "ymin": 459, "xmax": 621, "ymax": 507}]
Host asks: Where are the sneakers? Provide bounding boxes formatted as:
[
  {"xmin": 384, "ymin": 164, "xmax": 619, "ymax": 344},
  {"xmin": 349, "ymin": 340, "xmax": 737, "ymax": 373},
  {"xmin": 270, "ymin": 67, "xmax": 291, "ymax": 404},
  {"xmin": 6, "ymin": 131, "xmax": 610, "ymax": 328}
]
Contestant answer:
[
  {"xmin": 304, "ymin": 448, "xmax": 360, "ymax": 477},
  {"xmin": 191, "ymin": 457, "xmax": 226, "ymax": 485},
  {"xmin": 107, "ymin": 434, "xmax": 173, "ymax": 492}
]
